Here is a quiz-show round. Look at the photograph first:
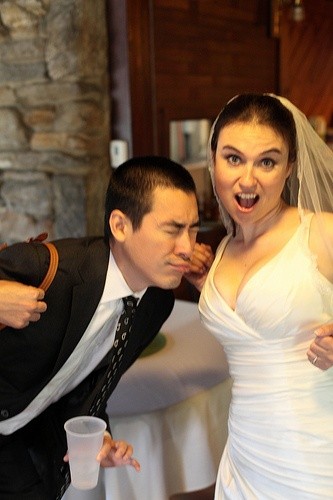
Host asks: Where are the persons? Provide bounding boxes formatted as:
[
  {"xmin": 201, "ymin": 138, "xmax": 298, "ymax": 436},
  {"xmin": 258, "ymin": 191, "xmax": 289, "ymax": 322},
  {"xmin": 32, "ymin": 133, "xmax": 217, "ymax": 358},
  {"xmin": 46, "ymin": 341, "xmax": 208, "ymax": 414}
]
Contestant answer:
[
  {"xmin": 0, "ymin": 155, "xmax": 199, "ymax": 500},
  {"xmin": 185, "ymin": 94, "xmax": 333, "ymax": 500}
]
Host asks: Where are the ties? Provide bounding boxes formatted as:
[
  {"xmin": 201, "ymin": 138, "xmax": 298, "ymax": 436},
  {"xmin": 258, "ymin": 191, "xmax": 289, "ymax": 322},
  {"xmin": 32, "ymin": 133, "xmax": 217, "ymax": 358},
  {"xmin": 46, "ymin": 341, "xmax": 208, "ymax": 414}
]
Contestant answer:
[{"xmin": 55, "ymin": 295, "xmax": 139, "ymax": 500}]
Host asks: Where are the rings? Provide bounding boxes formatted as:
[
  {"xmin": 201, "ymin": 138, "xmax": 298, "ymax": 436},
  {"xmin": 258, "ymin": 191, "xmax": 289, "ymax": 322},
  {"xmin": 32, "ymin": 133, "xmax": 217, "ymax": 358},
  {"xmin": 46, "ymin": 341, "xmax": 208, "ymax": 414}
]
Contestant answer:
[{"xmin": 313, "ymin": 356, "xmax": 318, "ymax": 364}]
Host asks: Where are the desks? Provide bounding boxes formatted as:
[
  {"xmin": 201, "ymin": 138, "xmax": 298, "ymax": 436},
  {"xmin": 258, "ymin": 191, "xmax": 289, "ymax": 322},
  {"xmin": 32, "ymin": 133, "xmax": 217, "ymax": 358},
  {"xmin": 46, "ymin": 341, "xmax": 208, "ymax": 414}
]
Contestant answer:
[{"xmin": 62, "ymin": 300, "xmax": 232, "ymax": 500}]
